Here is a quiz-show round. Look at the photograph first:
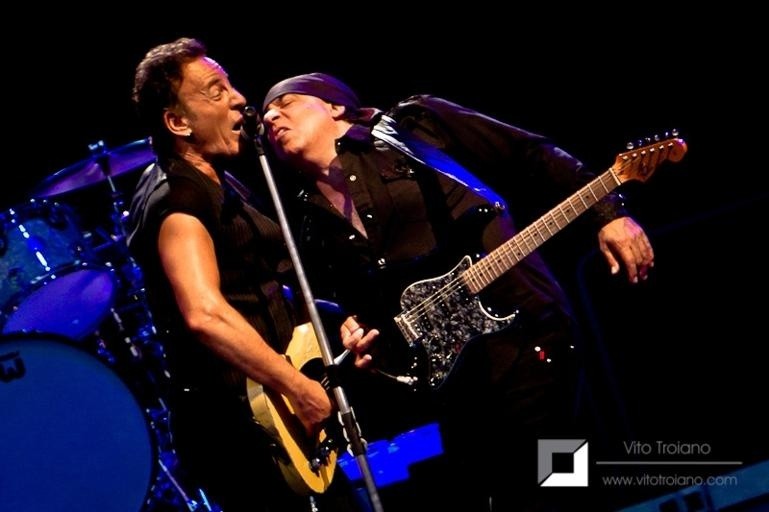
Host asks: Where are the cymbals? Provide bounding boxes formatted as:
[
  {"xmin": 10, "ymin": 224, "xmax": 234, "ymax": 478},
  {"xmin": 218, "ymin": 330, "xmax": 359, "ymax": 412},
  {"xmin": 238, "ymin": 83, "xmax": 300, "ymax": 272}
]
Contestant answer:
[{"xmin": 30, "ymin": 134, "xmax": 161, "ymax": 199}]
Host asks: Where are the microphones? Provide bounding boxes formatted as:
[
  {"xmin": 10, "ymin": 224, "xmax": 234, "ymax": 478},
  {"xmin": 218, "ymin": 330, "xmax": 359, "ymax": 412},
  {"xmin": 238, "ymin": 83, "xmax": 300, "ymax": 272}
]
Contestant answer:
[{"xmin": 241, "ymin": 107, "xmax": 265, "ymax": 138}]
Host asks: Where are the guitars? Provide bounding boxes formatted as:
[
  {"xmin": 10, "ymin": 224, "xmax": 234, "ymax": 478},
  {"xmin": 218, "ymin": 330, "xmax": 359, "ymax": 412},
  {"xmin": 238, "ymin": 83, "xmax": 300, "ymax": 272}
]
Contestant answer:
[
  {"xmin": 311, "ymin": 129, "xmax": 688, "ymax": 430},
  {"xmin": 246, "ymin": 320, "xmax": 357, "ymax": 498}
]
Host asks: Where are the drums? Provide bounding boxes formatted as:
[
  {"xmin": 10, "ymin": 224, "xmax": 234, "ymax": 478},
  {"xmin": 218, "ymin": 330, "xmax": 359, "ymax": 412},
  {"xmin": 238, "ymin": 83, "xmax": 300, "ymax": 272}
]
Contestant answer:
[
  {"xmin": 0, "ymin": 330, "xmax": 158, "ymax": 512},
  {"xmin": 1, "ymin": 199, "xmax": 119, "ymax": 343}
]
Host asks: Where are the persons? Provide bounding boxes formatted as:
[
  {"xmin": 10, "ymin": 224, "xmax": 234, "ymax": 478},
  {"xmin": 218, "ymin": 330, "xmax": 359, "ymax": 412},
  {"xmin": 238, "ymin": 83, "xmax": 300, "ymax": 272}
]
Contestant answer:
[
  {"xmin": 263, "ymin": 69, "xmax": 657, "ymax": 512},
  {"xmin": 126, "ymin": 37, "xmax": 370, "ymax": 511}
]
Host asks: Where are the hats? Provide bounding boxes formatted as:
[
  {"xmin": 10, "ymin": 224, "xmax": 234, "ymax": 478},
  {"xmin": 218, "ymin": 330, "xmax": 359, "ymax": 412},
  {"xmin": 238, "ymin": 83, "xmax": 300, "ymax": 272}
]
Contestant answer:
[{"xmin": 262, "ymin": 72, "xmax": 360, "ymax": 111}]
